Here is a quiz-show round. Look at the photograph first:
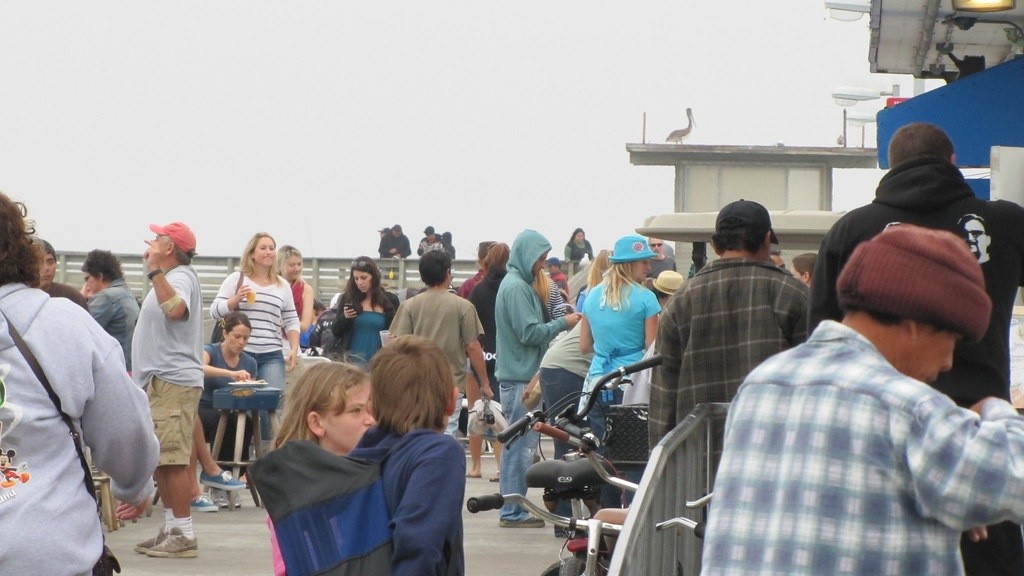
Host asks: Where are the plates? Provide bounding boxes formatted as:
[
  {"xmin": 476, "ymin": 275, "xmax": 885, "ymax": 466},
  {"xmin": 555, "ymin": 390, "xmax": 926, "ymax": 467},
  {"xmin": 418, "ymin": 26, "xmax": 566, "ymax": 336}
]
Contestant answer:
[{"xmin": 227, "ymin": 381, "xmax": 268, "ymax": 387}]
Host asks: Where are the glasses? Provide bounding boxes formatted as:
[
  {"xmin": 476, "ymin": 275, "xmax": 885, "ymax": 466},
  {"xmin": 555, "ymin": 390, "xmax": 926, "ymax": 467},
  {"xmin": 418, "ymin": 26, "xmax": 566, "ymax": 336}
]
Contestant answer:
[{"xmin": 649, "ymin": 243, "xmax": 663, "ymax": 247}]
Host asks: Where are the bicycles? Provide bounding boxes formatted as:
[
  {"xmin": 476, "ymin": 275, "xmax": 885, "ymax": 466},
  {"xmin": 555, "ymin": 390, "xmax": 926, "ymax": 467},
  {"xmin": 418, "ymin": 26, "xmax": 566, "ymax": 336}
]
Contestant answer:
[{"xmin": 463, "ymin": 353, "xmax": 713, "ymax": 576}]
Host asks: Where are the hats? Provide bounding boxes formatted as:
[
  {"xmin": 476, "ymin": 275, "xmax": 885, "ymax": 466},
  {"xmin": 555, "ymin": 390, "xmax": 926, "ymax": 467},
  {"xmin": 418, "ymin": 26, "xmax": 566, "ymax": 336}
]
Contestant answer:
[
  {"xmin": 713, "ymin": 199, "xmax": 777, "ymax": 241},
  {"xmin": 148, "ymin": 222, "xmax": 196, "ymax": 253},
  {"xmin": 653, "ymin": 270, "xmax": 683, "ymax": 295},
  {"xmin": 547, "ymin": 258, "xmax": 559, "ymax": 265},
  {"xmin": 837, "ymin": 222, "xmax": 994, "ymax": 339},
  {"xmin": 606, "ymin": 234, "xmax": 656, "ymax": 263}
]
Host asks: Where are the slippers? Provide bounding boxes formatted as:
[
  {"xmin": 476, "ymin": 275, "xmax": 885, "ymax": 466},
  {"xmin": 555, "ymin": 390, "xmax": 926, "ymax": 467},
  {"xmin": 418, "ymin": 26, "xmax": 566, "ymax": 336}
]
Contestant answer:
[{"xmin": 466, "ymin": 471, "xmax": 482, "ymax": 479}]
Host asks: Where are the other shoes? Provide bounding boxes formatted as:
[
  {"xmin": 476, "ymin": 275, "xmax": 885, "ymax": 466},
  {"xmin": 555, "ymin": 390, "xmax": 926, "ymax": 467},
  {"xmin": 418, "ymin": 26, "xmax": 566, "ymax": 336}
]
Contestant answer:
[{"xmin": 214, "ymin": 497, "xmax": 240, "ymax": 509}]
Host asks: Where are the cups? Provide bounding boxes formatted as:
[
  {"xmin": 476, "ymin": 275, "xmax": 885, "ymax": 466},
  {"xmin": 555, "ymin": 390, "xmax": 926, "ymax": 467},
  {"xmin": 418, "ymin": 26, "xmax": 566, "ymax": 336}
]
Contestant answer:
[
  {"xmin": 247, "ymin": 287, "xmax": 256, "ymax": 306},
  {"xmin": 380, "ymin": 330, "xmax": 392, "ymax": 348}
]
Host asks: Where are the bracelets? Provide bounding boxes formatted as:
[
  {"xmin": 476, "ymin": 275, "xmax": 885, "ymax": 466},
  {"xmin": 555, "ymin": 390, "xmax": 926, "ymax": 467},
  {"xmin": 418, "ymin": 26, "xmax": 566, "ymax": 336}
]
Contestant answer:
[{"xmin": 147, "ymin": 268, "xmax": 163, "ymax": 279}]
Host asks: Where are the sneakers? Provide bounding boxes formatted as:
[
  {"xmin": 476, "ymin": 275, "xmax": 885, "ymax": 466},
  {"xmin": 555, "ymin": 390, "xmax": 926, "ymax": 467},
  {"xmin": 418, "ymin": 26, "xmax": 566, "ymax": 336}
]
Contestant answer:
[
  {"xmin": 191, "ymin": 495, "xmax": 218, "ymax": 514},
  {"xmin": 200, "ymin": 470, "xmax": 246, "ymax": 491},
  {"xmin": 135, "ymin": 527, "xmax": 199, "ymax": 559},
  {"xmin": 500, "ymin": 515, "xmax": 545, "ymax": 530}
]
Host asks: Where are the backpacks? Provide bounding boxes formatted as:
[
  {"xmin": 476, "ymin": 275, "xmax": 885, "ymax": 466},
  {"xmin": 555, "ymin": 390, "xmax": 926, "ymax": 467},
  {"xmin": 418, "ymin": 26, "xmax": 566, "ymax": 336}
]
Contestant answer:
[{"xmin": 252, "ymin": 429, "xmax": 457, "ymax": 575}]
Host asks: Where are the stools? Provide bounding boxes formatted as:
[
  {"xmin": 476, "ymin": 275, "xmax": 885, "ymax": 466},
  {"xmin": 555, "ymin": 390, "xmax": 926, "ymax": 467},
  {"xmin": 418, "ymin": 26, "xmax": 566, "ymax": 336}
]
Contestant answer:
[{"xmin": 203, "ymin": 385, "xmax": 290, "ymax": 514}]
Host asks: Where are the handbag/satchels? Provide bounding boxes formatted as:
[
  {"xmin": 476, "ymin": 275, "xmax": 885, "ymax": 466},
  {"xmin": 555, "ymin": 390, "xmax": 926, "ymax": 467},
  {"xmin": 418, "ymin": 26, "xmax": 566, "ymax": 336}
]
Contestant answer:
[
  {"xmin": 92, "ymin": 545, "xmax": 120, "ymax": 575},
  {"xmin": 211, "ymin": 303, "xmax": 236, "ymax": 344}
]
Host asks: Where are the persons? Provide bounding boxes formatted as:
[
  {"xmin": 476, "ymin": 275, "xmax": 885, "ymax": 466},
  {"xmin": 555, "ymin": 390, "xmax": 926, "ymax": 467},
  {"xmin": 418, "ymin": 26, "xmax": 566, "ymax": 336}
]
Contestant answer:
[
  {"xmin": 351, "ymin": 334, "xmax": 466, "ymax": 575},
  {"xmin": 332, "ymin": 256, "xmax": 402, "ymax": 365},
  {"xmin": 699, "ymin": 224, "xmax": 1024, "ymax": 576},
  {"xmin": 132, "ymin": 221, "xmax": 200, "ymax": 556},
  {"xmin": 275, "ymin": 244, "xmax": 314, "ymax": 358},
  {"xmin": 1, "ymin": 190, "xmax": 161, "ymax": 576},
  {"xmin": 267, "ymin": 362, "xmax": 375, "ymax": 576},
  {"xmin": 812, "ymin": 125, "xmax": 1023, "ymax": 574},
  {"xmin": 299, "ymin": 294, "xmax": 343, "ymax": 347},
  {"xmin": 647, "ymin": 198, "xmax": 809, "ymax": 457},
  {"xmin": 202, "ymin": 312, "xmax": 256, "ymax": 508},
  {"xmin": 376, "ymin": 224, "xmax": 456, "ymax": 259},
  {"xmin": 770, "ymin": 253, "xmax": 817, "ymax": 289},
  {"xmin": 458, "ymin": 225, "xmax": 682, "ymax": 527},
  {"xmin": 27, "ymin": 235, "xmax": 245, "ymax": 514},
  {"xmin": 387, "ymin": 253, "xmax": 494, "ymax": 434},
  {"xmin": 210, "ymin": 231, "xmax": 300, "ymax": 438}
]
